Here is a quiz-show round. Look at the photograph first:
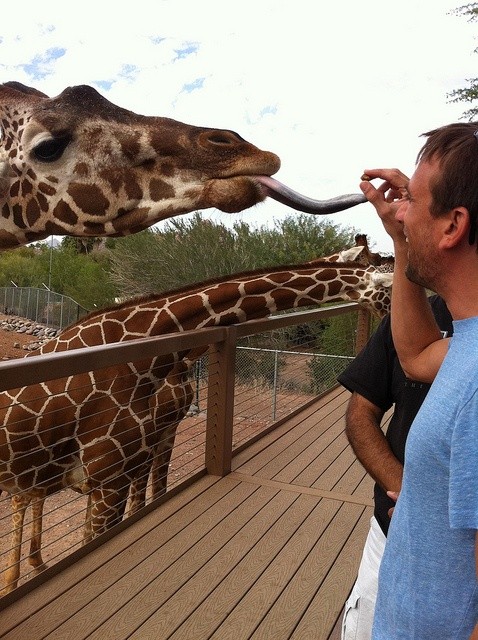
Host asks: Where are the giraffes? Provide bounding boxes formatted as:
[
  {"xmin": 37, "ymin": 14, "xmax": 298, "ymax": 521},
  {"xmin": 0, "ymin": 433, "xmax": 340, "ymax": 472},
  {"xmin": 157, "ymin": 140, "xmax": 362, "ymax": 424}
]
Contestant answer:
[
  {"xmin": 1, "ymin": 79, "xmax": 373, "ymax": 251},
  {"xmin": 2, "ymin": 233, "xmax": 398, "ymax": 589}
]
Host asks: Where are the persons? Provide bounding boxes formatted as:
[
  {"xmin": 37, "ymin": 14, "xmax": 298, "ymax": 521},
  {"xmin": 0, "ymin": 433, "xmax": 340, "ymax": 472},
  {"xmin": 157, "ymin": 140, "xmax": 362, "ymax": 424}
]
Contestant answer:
[
  {"xmin": 360, "ymin": 122, "xmax": 478, "ymax": 640},
  {"xmin": 337, "ymin": 294, "xmax": 453, "ymax": 640}
]
